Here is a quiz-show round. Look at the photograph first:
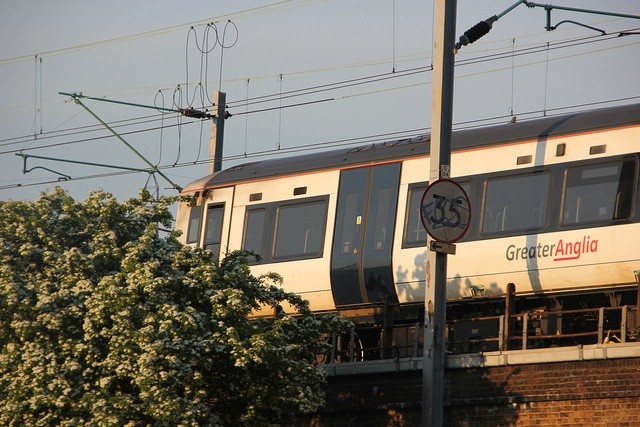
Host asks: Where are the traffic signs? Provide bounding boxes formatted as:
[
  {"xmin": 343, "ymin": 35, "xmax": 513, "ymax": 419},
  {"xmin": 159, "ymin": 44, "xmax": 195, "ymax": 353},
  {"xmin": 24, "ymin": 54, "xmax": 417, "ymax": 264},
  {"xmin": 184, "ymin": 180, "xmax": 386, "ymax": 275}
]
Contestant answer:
[{"xmin": 420, "ymin": 179, "xmax": 472, "ymax": 244}]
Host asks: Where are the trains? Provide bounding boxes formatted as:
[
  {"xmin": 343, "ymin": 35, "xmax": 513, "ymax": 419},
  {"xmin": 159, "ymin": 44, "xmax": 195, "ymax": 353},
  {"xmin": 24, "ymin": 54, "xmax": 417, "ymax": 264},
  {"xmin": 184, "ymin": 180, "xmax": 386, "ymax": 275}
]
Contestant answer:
[{"xmin": 172, "ymin": 102, "xmax": 640, "ymax": 365}]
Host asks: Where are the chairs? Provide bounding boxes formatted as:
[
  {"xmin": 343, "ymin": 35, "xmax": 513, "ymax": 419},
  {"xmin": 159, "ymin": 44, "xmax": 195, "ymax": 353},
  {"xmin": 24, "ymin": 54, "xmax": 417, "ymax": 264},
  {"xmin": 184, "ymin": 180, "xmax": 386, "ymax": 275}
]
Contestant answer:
[
  {"xmin": 536, "ymin": 202, "xmax": 545, "ymax": 226},
  {"xmin": 302, "ymin": 227, "xmax": 322, "ymax": 253},
  {"xmin": 573, "ymin": 197, "xmax": 597, "ymax": 223},
  {"xmin": 501, "ymin": 205, "xmax": 527, "ymax": 232}
]
